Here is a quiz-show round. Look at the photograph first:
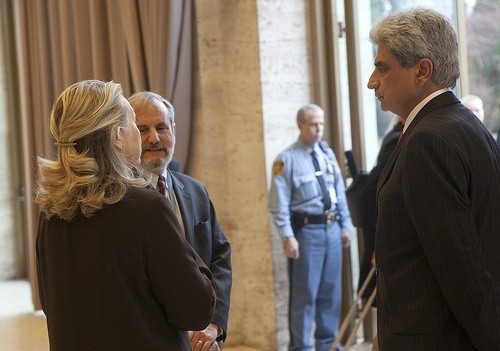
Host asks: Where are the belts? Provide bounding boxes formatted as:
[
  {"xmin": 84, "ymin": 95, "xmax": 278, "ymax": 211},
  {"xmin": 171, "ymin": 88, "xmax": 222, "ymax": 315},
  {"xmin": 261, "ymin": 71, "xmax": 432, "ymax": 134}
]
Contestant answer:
[{"xmin": 304, "ymin": 211, "xmax": 339, "ymax": 224}]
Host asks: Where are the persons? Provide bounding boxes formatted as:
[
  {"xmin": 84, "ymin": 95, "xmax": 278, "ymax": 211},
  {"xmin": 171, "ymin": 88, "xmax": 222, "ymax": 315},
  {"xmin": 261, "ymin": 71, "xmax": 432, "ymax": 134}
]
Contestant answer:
[
  {"xmin": 367, "ymin": 7, "xmax": 500, "ymax": 351},
  {"xmin": 35, "ymin": 80, "xmax": 217, "ymax": 351},
  {"xmin": 359, "ymin": 95, "xmax": 485, "ymax": 309},
  {"xmin": 269, "ymin": 103, "xmax": 354, "ymax": 351},
  {"xmin": 127, "ymin": 87, "xmax": 233, "ymax": 351}
]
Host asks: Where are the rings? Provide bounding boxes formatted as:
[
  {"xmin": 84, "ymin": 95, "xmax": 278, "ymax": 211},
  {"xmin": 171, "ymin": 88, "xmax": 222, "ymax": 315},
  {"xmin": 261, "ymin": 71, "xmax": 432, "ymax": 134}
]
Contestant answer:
[{"xmin": 198, "ymin": 340, "xmax": 203, "ymax": 345}]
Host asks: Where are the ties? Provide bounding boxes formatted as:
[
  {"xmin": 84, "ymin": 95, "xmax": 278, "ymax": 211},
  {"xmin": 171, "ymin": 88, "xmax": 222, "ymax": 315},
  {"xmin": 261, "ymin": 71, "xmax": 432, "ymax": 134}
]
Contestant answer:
[
  {"xmin": 309, "ymin": 151, "xmax": 332, "ymax": 210},
  {"xmin": 157, "ymin": 174, "xmax": 171, "ymax": 207}
]
